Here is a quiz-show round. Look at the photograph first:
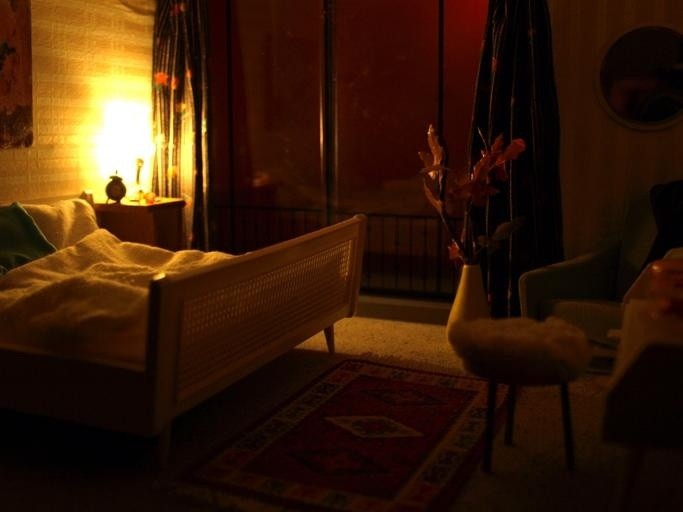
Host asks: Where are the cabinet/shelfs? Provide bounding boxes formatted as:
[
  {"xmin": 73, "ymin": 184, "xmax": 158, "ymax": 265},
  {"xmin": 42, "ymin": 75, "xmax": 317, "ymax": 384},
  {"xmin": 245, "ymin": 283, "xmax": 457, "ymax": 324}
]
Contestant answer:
[{"xmin": 204, "ymin": 0, "xmax": 489, "ymax": 263}]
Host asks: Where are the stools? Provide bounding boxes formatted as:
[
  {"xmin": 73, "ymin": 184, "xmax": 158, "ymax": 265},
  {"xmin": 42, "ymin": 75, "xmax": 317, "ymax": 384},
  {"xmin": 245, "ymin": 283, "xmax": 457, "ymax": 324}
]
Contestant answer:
[{"xmin": 452, "ymin": 315, "xmax": 593, "ymax": 475}]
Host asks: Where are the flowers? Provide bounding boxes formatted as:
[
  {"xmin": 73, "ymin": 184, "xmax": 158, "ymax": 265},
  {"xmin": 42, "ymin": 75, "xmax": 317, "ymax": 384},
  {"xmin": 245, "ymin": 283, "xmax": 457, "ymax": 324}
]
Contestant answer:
[{"xmin": 417, "ymin": 119, "xmax": 525, "ymax": 264}]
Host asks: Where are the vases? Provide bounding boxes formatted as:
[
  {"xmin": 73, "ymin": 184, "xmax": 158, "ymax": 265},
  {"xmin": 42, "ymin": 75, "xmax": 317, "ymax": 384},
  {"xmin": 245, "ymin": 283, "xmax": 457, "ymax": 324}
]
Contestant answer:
[{"xmin": 447, "ymin": 263, "xmax": 491, "ymax": 357}]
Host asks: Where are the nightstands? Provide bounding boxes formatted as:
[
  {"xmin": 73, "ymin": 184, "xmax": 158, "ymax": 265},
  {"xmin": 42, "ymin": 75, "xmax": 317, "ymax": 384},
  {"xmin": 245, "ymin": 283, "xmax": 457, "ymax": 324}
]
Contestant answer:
[{"xmin": 92, "ymin": 195, "xmax": 188, "ymax": 251}]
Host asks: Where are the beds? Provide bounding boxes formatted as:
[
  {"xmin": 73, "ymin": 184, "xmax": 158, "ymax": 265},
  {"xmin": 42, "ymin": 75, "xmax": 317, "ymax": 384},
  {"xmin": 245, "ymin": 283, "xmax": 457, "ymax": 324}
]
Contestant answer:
[{"xmin": 0, "ymin": 212, "xmax": 368, "ymax": 470}]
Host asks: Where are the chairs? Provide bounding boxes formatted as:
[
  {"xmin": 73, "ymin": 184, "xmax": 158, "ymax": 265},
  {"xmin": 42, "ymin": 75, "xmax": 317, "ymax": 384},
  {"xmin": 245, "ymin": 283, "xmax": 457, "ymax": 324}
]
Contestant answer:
[{"xmin": 517, "ymin": 192, "xmax": 683, "ymax": 375}]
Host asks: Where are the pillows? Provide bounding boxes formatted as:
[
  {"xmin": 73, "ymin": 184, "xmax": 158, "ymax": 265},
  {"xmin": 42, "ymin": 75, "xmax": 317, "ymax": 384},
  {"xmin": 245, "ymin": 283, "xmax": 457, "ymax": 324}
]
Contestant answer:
[
  {"xmin": 0, "ymin": 202, "xmax": 56, "ymax": 280},
  {"xmin": 19, "ymin": 196, "xmax": 100, "ymax": 251}
]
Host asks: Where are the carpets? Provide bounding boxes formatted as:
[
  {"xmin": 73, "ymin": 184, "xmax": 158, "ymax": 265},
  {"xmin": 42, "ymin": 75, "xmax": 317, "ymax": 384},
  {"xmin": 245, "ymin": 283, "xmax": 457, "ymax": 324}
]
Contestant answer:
[{"xmin": 170, "ymin": 358, "xmax": 523, "ymax": 512}]
